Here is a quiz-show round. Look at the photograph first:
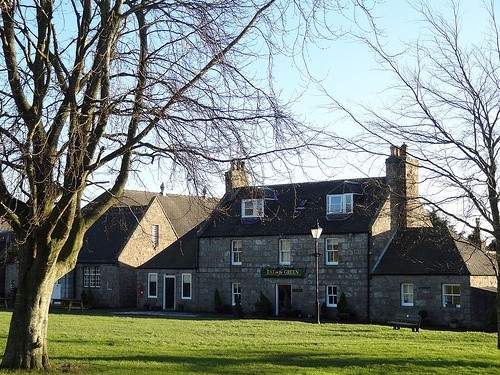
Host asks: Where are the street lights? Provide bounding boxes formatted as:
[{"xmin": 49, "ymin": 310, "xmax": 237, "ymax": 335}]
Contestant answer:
[{"xmin": 310, "ymin": 218, "xmax": 324, "ymax": 324}]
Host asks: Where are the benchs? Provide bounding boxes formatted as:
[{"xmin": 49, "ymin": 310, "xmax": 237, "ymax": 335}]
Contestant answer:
[
  {"xmin": 0, "ymin": 298, "xmax": 15, "ymax": 312},
  {"xmin": 51, "ymin": 298, "xmax": 87, "ymax": 315},
  {"xmin": 386, "ymin": 313, "xmax": 421, "ymax": 332}
]
